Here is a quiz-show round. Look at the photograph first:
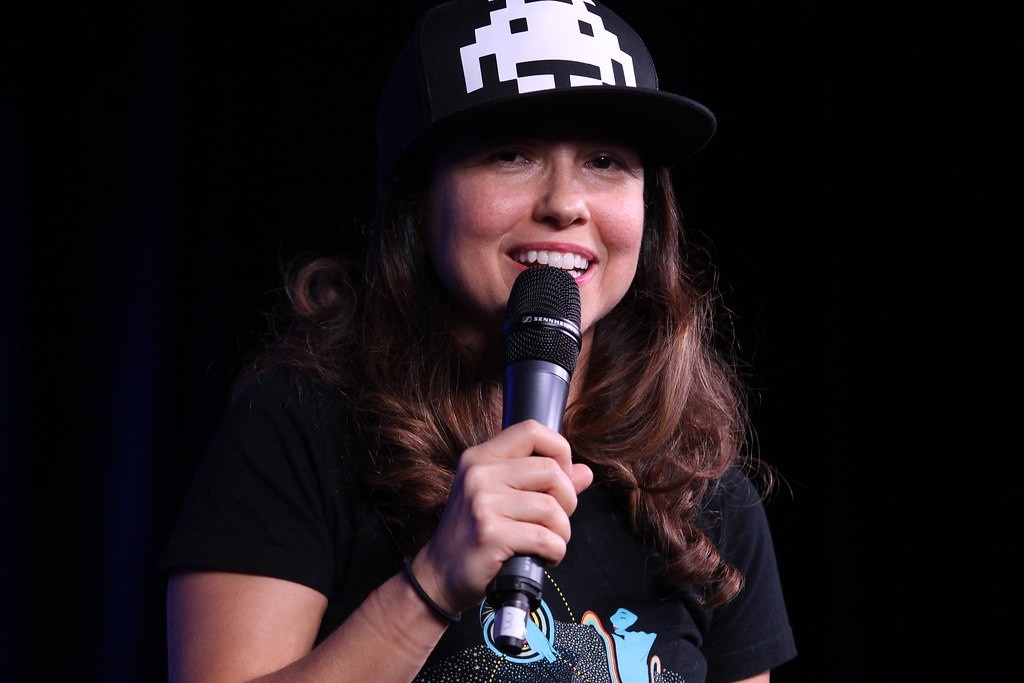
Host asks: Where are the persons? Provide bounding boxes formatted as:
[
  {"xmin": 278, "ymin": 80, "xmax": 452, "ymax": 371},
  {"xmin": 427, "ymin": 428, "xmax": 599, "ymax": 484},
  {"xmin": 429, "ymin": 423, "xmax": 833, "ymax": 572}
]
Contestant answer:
[{"xmin": 158, "ymin": 0, "xmax": 799, "ymax": 682}]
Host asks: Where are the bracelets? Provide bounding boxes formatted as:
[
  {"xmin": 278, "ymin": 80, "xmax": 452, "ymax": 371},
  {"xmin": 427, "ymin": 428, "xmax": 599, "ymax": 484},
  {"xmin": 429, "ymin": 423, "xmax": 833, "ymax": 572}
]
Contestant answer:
[{"xmin": 399, "ymin": 556, "xmax": 465, "ymax": 624}]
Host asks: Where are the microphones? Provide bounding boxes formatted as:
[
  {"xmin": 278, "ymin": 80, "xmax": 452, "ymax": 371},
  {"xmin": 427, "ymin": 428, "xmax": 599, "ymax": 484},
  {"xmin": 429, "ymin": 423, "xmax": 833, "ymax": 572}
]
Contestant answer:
[{"xmin": 488, "ymin": 264, "xmax": 582, "ymax": 658}]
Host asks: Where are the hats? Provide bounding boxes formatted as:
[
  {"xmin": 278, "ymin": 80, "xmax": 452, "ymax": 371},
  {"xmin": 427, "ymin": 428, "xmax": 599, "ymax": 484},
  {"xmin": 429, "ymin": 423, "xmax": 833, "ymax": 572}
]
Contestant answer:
[{"xmin": 367, "ymin": 0, "xmax": 717, "ymax": 179}]
